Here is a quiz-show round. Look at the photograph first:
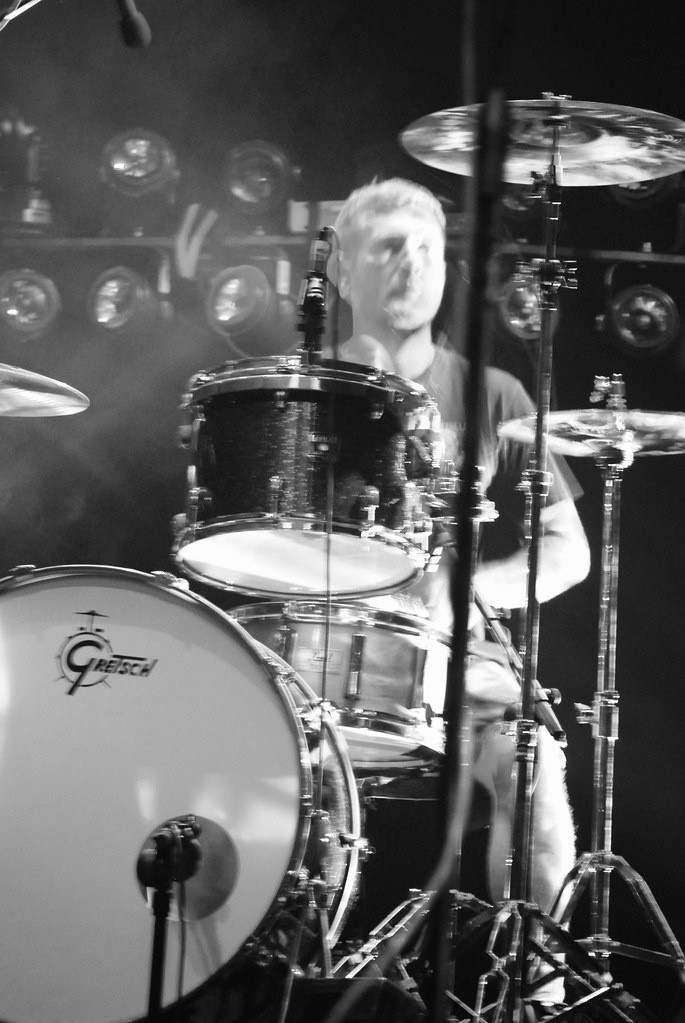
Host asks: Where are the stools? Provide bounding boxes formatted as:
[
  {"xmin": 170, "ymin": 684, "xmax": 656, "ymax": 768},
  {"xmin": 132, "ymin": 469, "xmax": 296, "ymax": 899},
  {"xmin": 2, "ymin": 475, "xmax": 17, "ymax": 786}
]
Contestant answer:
[{"xmin": 323, "ymin": 777, "xmax": 505, "ymax": 1023}]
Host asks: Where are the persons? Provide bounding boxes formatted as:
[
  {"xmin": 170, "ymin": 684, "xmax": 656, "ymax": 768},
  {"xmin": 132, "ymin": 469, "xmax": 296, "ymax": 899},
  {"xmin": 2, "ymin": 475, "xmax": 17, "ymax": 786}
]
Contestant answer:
[{"xmin": 326, "ymin": 178, "xmax": 591, "ymax": 1023}]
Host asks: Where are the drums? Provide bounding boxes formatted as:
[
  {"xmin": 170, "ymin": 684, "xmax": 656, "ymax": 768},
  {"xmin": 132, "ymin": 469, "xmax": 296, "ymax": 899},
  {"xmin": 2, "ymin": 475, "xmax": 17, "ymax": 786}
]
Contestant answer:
[
  {"xmin": 0, "ymin": 559, "xmax": 366, "ymax": 1023},
  {"xmin": 223, "ymin": 598, "xmax": 458, "ymax": 780},
  {"xmin": 172, "ymin": 352, "xmax": 445, "ymax": 602}
]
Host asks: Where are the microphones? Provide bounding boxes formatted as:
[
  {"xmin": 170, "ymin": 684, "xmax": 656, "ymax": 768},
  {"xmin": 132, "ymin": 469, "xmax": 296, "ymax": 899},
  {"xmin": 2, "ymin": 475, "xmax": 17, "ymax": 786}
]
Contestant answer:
[
  {"xmin": 116, "ymin": 0, "xmax": 153, "ymax": 50},
  {"xmin": 301, "ymin": 228, "xmax": 331, "ymax": 365},
  {"xmin": 137, "ymin": 836, "xmax": 201, "ymax": 917}
]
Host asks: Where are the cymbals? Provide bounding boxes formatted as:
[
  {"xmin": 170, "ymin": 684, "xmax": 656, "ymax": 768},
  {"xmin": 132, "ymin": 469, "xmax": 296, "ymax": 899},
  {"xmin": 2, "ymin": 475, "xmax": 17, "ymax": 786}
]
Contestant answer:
[
  {"xmin": 394, "ymin": 91, "xmax": 683, "ymax": 192},
  {"xmin": 495, "ymin": 392, "xmax": 685, "ymax": 467},
  {"xmin": 0, "ymin": 356, "xmax": 89, "ymax": 423}
]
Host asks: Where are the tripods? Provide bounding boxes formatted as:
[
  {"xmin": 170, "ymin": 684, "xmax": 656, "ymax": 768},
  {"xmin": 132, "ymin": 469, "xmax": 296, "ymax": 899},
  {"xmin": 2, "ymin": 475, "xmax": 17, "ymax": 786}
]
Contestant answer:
[{"xmin": 317, "ymin": 136, "xmax": 685, "ymax": 1023}]
